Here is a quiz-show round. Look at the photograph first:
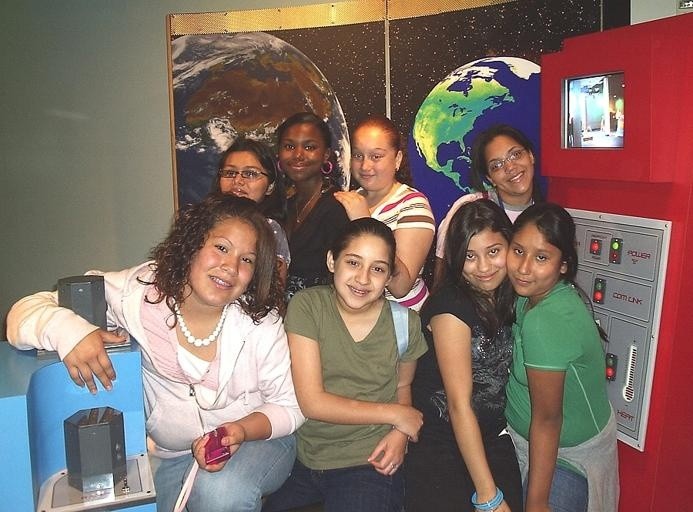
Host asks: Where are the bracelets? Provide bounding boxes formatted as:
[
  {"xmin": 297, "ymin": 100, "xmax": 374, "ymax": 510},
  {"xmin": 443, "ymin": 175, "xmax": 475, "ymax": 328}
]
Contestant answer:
[{"xmin": 467, "ymin": 487, "xmax": 504, "ymax": 510}]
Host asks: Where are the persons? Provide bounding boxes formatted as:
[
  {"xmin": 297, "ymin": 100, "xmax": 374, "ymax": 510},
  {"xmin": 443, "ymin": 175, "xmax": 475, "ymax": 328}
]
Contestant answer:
[
  {"xmin": 213, "ymin": 137, "xmax": 291, "ymax": 298},
  {"xmin": 261, "ymin": 218, "xmax": 429, "ymax": 512},
  {"xmin": 335, "ymin": 115, "xmax": 435, "ymax": 312},
  {"xmin": 427, "ymin": 125, "xmax": 538, "ymax": 298},
  {"xmin": 504, "ymin": 203, "xmax": 620, "ymax": 510},
  {"xmin": 5, "ymin": 191, "xmax": 308, "ymax": 512},
  {"xmin": 273, "ymin": 113, "xmax": 350, "ymax": 308},
  {"xmin": 402, "ymin": 198, "xmax": 522, "ymax": 511}
]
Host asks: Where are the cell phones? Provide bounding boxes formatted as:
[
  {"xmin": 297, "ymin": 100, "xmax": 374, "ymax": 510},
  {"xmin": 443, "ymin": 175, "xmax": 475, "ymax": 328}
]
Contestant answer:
[{"xmin": 203, "ymin": 427, "xmax": 231, "ymax": 465}]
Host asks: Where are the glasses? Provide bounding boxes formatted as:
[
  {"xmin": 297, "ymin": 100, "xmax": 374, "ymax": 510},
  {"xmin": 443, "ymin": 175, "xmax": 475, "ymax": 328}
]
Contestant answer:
[
  {"xmin": 218, "ymin": 168, "xmax": 269, "ymax": 179},
  {"xmin": 489, "ymin": 148, "xmax": 527, "ymax": 172}
]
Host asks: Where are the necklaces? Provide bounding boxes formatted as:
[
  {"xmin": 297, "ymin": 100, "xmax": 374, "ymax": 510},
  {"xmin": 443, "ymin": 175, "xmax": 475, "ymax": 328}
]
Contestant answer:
[
  {"xmin": 293, "ymin": 182, "xmax": 324, "ymax": 223},
  {"xmin": 168, "ymin": 296, "xmax": 232, "ymax": 346}
]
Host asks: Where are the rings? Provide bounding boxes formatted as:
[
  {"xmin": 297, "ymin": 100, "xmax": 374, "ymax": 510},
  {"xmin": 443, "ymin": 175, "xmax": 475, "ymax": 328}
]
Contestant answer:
[{"xmin": 390, "ymin": 460, "xmax": 396, "ymax": 468}]
